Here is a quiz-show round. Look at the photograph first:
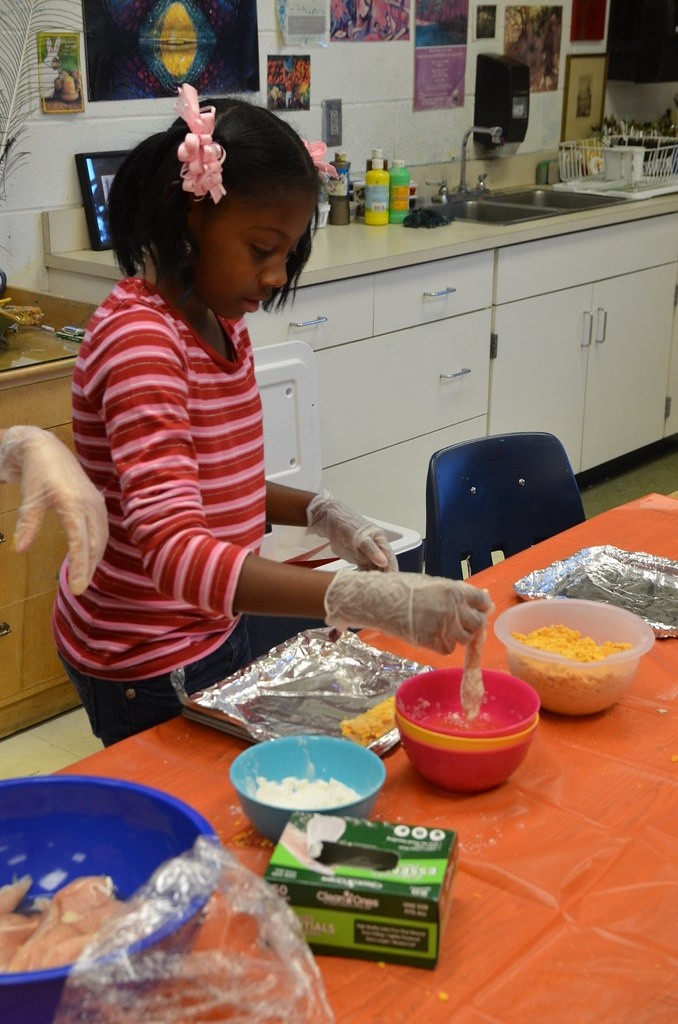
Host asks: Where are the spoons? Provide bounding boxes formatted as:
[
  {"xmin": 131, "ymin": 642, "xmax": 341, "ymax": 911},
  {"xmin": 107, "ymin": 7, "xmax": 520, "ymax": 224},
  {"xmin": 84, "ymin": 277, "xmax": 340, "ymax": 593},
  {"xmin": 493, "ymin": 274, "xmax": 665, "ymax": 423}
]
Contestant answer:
[{"xmin": 459, "ymin": 588, "xmax": 485, "ymax": 711}]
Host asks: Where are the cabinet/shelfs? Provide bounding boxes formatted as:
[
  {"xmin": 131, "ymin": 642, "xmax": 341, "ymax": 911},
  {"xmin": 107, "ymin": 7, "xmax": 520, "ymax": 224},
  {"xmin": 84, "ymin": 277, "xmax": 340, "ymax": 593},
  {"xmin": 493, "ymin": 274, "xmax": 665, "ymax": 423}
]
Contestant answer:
[
  {"xmin": 245, "ymin": 211, "xmax": 678, "ymax": 562},
  {"xmin": 1, "ymin": 284, "xmax": 100, "ymax": 739}
]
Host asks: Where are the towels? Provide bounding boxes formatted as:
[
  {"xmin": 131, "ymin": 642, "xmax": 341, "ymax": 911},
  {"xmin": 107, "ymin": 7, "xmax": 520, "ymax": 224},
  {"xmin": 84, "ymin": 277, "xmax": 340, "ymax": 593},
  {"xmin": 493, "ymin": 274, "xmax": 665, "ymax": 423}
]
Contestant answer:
[{"xmin": 403, "ymin": 207, "xmax": 455, "ymax": 229}]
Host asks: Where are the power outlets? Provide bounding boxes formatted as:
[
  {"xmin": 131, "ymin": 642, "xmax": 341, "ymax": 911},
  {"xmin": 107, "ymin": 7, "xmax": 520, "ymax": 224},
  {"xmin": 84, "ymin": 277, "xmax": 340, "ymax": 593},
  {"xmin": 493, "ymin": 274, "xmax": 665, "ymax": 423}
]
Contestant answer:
[{"xmin": 322, "ymin": 98, "xmax": 342, "ymax": 148}]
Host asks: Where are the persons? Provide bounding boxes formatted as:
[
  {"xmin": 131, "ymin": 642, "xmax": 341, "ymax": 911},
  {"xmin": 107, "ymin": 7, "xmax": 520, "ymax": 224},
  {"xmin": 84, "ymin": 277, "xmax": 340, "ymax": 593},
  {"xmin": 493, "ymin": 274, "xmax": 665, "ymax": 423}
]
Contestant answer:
[
  {"xmin": 50, "ymin": 99, "xmax": 492, "ymax": 747},
  {"xmin": 0, "ymin": 424, "xmax": 108, "ymax": 596}
]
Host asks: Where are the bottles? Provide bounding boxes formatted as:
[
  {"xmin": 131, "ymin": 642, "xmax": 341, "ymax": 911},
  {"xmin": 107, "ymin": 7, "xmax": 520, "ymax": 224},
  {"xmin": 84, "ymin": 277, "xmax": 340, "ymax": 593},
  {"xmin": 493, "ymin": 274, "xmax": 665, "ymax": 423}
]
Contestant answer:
[
  {"xmin": 365, "ymin": 148, "xmax": 388, "ymax": 171},
  {"xmin": 364, "ymin": 158, "xmax": 389, "ymax": 225},
  {"xmin": 388, "ymin": 160, "xmax": 410, "ymax": 223},
  {"xmin": 328, "ymin": 151, "xmax": 351, "ymax": 225}
]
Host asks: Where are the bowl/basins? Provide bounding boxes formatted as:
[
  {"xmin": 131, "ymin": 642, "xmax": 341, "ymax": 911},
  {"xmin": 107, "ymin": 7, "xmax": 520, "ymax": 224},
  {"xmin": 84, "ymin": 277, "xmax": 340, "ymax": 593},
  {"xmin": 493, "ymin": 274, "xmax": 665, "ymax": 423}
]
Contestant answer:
[
  {"xmin": 0, "ymin": 775, "xmax": 221, "ymax": 1024},
  {"xmin": 494, "ymin": 597, "xmax": 655, "ymax": 716},
  {"xmin": 395, "ymin": 667, "xmax": 541, "ymax": 794},
  {"xmin": 228, "ymin": 733, "xmax": 385, "ymax": 846}
]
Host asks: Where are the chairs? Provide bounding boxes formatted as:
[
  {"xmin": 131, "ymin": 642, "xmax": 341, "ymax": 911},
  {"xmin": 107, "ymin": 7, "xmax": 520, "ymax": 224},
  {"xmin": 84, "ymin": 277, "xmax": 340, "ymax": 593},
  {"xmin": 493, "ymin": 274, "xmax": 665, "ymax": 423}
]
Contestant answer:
[{"xmin": 427, "ymin": 432, "xmax": 587, "ymax": 581}]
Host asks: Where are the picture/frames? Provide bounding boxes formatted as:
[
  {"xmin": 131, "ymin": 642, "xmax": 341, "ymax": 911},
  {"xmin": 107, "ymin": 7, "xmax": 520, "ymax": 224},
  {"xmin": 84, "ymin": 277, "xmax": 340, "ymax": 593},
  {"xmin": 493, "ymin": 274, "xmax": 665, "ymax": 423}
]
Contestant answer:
[
  {"xmin": 75, "ymin": 149, "xmax": 135, "ymax": 252},
  {"xmin": 560, "ymin": 52, "xmax": 609, "ymax": 148}
]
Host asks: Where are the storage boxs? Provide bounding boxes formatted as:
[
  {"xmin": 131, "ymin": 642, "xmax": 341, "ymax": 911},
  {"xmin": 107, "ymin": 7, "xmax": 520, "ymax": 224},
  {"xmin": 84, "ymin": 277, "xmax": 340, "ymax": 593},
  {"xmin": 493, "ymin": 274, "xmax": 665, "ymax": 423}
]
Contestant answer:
[
  {"xmin": 245, "ymin": 342, "xmax": 426, "ymax": 644},
  {"xmin": 263, "ymin": 813, "xmax": 460, "ymax": 970}
]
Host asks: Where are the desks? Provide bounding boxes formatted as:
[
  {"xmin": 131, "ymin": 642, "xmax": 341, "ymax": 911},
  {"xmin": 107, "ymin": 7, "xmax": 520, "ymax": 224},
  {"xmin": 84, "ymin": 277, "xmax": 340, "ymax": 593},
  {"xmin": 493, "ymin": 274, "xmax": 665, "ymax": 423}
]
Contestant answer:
[{"xmin": 53, "ymin": 491, "xmax": 678, "ymax": 1024}]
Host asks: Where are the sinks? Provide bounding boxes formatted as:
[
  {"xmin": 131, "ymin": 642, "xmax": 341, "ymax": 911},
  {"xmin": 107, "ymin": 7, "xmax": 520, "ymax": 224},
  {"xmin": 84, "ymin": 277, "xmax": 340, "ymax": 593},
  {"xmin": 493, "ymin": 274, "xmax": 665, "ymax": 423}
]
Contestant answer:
[
  {"xmin": 410, "ymin": 193, "xmax": 570, "ymax": 225},
  {"xmin": 474, "ymin": 187, "xmax": 649, "ymax": 211}
]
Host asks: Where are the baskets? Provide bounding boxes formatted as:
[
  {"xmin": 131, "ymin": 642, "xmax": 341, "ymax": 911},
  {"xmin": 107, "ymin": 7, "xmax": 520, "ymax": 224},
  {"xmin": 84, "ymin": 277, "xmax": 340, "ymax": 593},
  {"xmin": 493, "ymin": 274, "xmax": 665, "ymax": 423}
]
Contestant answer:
[{"xmin": 558, "ymin": 135, "xmax": 678, "ymax": 191}]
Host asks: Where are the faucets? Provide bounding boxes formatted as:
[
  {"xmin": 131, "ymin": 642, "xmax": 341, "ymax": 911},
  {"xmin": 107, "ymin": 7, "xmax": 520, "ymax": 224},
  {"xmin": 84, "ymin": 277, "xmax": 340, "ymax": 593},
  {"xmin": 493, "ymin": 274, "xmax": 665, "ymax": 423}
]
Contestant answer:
[{"xmin": 425, "ymin": 126, "xmax": 505, "ymax": 205}]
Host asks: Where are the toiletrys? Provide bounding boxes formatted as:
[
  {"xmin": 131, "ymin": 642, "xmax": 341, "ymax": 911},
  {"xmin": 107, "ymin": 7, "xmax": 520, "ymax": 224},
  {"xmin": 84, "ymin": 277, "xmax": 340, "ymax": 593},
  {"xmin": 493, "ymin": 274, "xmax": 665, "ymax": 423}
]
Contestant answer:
[
  {"xmin": 387, "ymin": 159, "xmax": 410, "ymax": 225},
  {"xmin": 364, "ymin": 158, "xmax": 390, "ymax": 225},
  {"xmin": 328, "ymin": 151, "xmax": 351, "ymax": 226},
  {"xmin": 366, "ymin": 148, "xmax": 388, "ymax": 173}
]
